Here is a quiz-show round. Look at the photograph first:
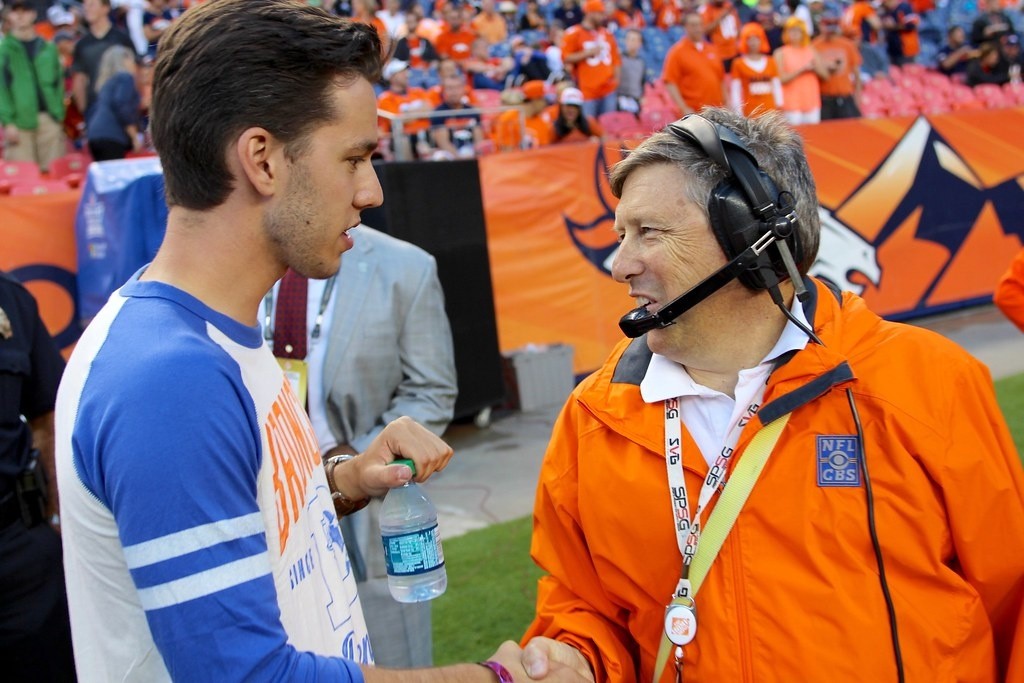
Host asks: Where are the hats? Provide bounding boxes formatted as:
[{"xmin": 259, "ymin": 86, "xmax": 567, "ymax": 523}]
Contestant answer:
[
  {"xmin": 381, "ymin": 57, "xmax": 408, "ymax": 80},
  {"xmin": 559, "ymin": 87, "xmax": 584, "ymax": 107},
  {"xmin": 581, "ymin": 0, "xmax": 604, "ymax": 11}
]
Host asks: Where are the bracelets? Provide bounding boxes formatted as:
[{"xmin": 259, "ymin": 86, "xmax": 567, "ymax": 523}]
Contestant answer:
[{"xmin": 478, "ymin": 660, "xmax": 515, "ymax": 683}]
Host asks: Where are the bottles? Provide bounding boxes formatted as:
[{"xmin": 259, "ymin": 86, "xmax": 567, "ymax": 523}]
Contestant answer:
[{"xmin": 378, "ymin": 460, "xmax": 447, "ymax": 602}]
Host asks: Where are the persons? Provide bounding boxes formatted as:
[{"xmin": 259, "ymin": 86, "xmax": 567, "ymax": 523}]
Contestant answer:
[
  {"xmin": 517, "ymin": 107, "xmax": 1023, "ymax": 683},
  {"xmin": 53, "ymin": 0, "xmax": 597, "ymax": 683},
  {"xmin": 0, "ymin": 2, "xmax": 1024, "ymax": 161},
  {"xmin": 262, "ymin": 217, "xmax": 460, "ymax": 668}
]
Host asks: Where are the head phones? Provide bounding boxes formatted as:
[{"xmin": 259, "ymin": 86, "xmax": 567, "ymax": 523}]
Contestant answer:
[{"xmin": 664, "ymin": 114, "xmax": 810, "ymax": 302}]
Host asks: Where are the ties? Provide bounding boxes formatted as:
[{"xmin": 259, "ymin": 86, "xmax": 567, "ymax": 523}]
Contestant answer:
[{"xmin": 273, "ymin": 266, "xmax": 309, "ymax": 419}]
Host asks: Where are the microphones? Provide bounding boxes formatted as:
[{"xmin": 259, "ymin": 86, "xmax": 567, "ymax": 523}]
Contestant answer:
[{"xmin": 618, "ymin": 217, "xmax": 793, "ymax": 339}]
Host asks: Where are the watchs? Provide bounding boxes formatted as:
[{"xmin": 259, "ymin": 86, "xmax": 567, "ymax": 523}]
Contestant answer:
[{"xmin": 324, "ymin": 454, "xmax": 372, "ymax": 516}]
